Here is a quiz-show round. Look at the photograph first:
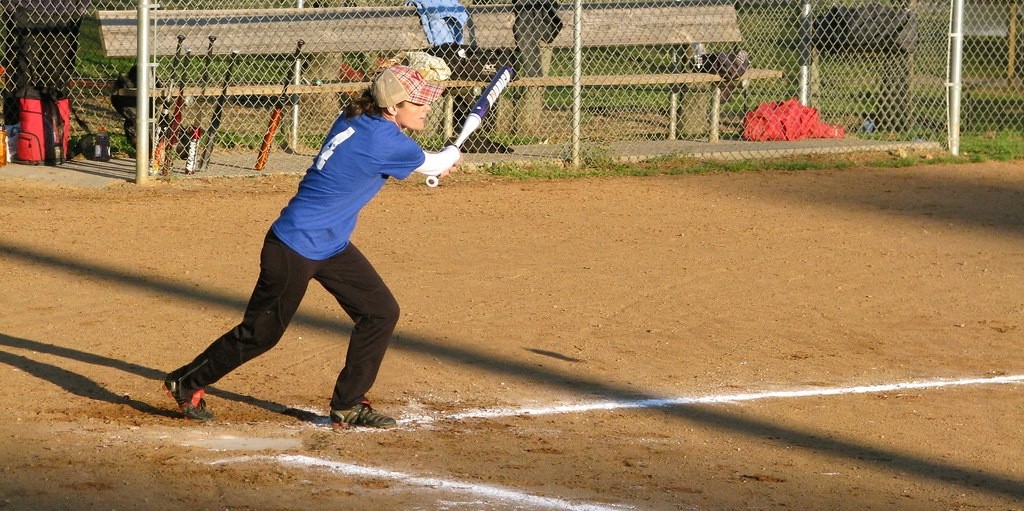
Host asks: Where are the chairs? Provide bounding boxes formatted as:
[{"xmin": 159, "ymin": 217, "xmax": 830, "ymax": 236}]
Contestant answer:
[{"xmin": 776, "ymin": 0, "xmax": 919, "ymax": 130}]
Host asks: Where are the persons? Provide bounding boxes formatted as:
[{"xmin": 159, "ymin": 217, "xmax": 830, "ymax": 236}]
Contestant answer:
[{"xmin": 165, "ymin": 65, "xmax": 463, "ymax": 429}]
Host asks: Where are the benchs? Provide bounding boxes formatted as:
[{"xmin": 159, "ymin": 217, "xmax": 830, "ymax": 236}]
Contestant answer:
[{"xmin": 95, "ymin": 4, "xmax": 782, "ymax": 150}]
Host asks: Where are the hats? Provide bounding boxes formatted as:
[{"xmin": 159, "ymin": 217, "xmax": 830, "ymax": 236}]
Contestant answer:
[{"xmin": 370, "ymin": 65, "xmax": 445, "ymax": 108}]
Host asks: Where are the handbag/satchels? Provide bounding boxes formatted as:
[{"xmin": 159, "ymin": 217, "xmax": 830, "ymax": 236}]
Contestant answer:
[
  {"xmin": 744, "ymin": 98, "xmax": 844, "ymax": 141},
  {"xmin": 691, "ymin": 48, "xmax": 749, "ymax": 79}
]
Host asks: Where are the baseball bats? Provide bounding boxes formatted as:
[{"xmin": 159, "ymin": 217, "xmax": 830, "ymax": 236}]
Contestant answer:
[
  {"xmin": 255, "ymin": 39, "xmax": 305, "ymax": 170},
  {"xmin": 425, "ymin": 65, "xmax": 514, "ymax": 188},
  {"xmin": 152, "ymin": 35, "xmax": 185, "ymax": 173},
  {"xmin": 162, "ymin": 49, "xmax": 191, "ymax": 174},
  {"xmin": 198, "ymin": 51, "xmax": 239, "ymax": 172},
  {"xmin": 185, "ymin": 36, "xmax": 217, "ymax": 174}
]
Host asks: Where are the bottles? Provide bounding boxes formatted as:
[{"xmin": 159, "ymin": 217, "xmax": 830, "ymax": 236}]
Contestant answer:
[{"xmin": 93, "ymin": 129, "xmax": 111, "ymax": 162}]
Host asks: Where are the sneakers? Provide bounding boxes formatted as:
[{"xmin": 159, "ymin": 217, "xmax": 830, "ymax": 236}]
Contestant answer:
[
  {"xmin": 162, "ymin": 373, "xmax": 213, "ymax": 421},
  {"xmin": 330, "ymin": 399, "xmax": 397, "ymax": 429}
]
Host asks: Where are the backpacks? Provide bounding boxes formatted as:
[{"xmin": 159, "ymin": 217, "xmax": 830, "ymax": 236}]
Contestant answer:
[{"xmin": 16, "ymin": 86, "xmax": 71, "ymax": 163}]
